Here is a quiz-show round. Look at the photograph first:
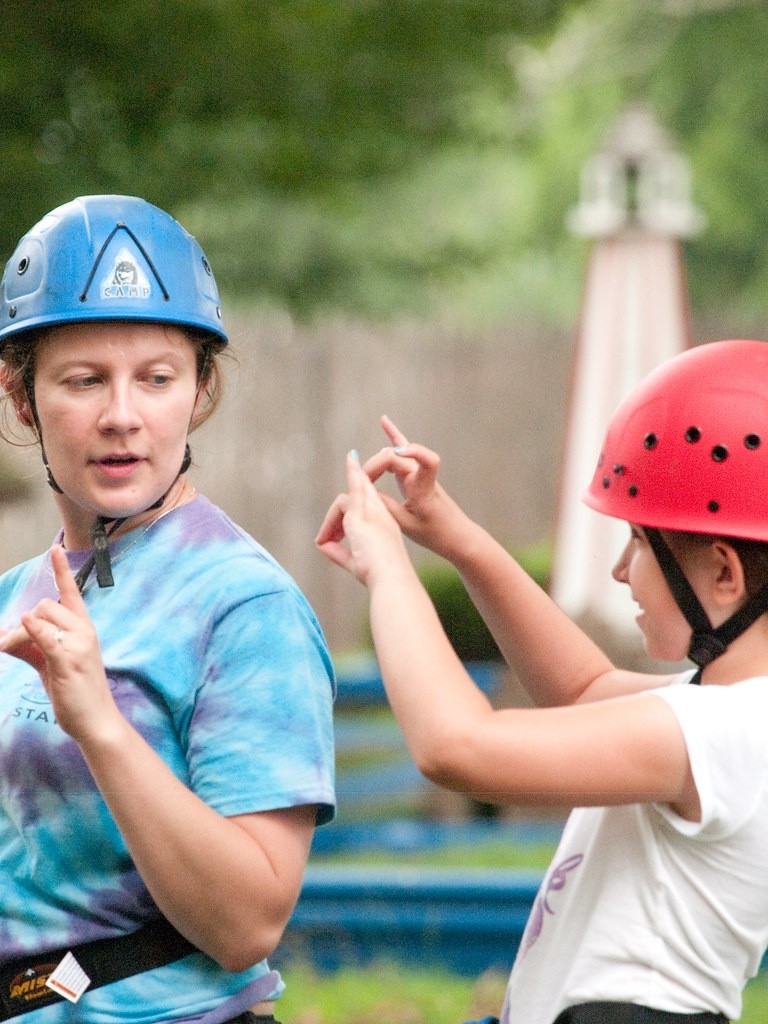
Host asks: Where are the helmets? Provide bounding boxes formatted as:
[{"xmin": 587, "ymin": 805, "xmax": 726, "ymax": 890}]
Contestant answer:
[
  {"xmin": 0, "ymin": 195, "xmax": 229, "ymax": 360},
  {"xmin": 583, "ymin": 338, "xmax": 768, "ymax": 542}
]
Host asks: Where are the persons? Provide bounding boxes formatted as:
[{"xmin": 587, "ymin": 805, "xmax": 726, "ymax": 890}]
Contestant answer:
[
  {"xmin": 0, "ymin": 192, "xmax": 342, "ymax": 1024},
  {"xmin": 315, "ymin": 339, "xmax": 768, "ymax": 1024}
]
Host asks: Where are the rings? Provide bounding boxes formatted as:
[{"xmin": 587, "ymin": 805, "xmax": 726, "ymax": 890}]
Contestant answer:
[{"xmin": 55, "ymin": 629, "xmax": 64, "ymax": 641}]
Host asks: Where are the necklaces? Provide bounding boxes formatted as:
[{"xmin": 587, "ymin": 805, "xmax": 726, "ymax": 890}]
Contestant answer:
[{"xmin": 53, "ymin": 488, "xmax": 195, "ymax": 592}]
